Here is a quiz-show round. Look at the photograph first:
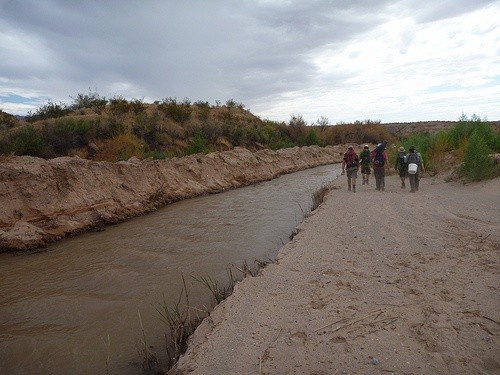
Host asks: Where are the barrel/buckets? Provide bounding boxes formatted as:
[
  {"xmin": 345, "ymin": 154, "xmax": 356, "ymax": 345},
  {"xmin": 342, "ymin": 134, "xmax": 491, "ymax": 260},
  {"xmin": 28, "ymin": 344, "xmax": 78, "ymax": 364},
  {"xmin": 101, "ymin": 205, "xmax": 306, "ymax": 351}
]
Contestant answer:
[{"xmin": 408, "ymin": 163, "xmax": 418, "ymax": 175}]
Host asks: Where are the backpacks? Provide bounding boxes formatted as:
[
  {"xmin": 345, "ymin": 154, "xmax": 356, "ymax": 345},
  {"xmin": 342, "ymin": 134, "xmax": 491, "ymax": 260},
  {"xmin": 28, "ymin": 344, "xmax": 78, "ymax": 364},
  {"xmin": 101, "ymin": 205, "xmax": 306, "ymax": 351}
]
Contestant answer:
[
  {"xmin": 345, "ymin": 153, "xmax": 358, "ymax": 167},
  {"xmin": 399, "ymin": 155, "xmax": 406, "ymax": 165},
  {"xmin": 363, "ymin": 154, "xmax": 370, "ymax": 164},
  {"xmin": 408, "ymin": 163, "xmax": 418, "ymax": 175},
  {"xmin": 374, "ymin": 154, "xmax": 385, "ymax": 167}
]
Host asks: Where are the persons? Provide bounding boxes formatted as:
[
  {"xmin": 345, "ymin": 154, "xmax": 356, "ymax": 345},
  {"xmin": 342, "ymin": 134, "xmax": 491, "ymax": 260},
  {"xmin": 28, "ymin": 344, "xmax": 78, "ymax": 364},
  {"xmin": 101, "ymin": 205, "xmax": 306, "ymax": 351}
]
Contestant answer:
[
  {"xmin": 368, "ymin": 143, "xmax": 390, "ymax": 191},
  {"xmin": 343, "ymin": 145, "xmax": 358, "ymax": 193},
  {"xmin": 406, "ymin": 145, "xmax": 425, "ymax": 192},
  {"xmin": 395, "ymin": 147, "xmax": 408, "ymax": 187},
  {"xmin": 358, "ymin": 144, "xmax": 372, "ymax": 184}
]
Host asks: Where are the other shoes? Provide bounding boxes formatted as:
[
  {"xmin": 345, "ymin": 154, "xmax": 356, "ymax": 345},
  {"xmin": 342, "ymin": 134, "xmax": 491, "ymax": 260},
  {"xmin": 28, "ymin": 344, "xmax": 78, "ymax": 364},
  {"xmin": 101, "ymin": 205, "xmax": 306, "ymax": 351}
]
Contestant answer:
[
  {"xmin": 362, "ymin": 180, "xmax": 369, "ymax": 186},
  {"xmin": 400, "ymin": 183, "xmax": 406, "ymax": 189},
  {"xmin": 349, "ymin": 185, "xmax": 356, "ymax": 192},
  {"xmin": 410, "ymin": 188, "xmax": 420, "ymax": 193},
  {"xmin": 377, "ymin": 186, "xmax": 385, "ymax": 192}
]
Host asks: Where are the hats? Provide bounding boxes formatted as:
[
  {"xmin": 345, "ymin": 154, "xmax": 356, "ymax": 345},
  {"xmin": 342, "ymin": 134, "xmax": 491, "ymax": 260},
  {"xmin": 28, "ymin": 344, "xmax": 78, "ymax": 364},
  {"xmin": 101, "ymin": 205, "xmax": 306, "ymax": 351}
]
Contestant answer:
[
  {"xmin": 376, "ymin": 143, "xmax": 386, "ymax": 149},
  {"xmin": 364, "ymin": 145, "xmax": 369, "ymax": 148},
  {"xmin": 348, "ymin": 145, "xmax": 354, "ymax": 149},
  {"xmin": 409, "ymin": 145, "xmax": 417, "ymax": 150},
  {"xmin": 398, "ymin": 147, "xmax": 405, "ymax": 152}
]
